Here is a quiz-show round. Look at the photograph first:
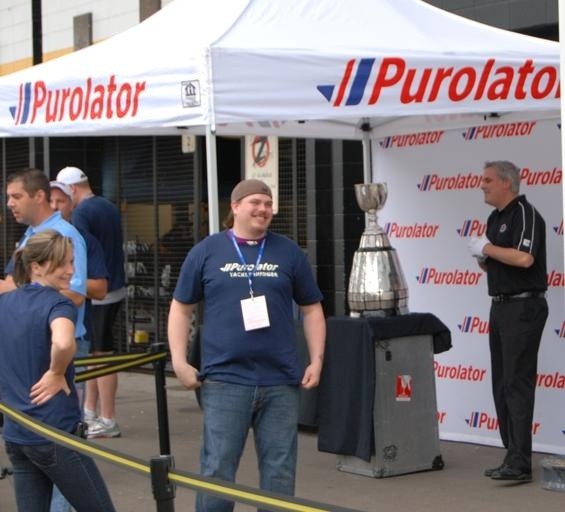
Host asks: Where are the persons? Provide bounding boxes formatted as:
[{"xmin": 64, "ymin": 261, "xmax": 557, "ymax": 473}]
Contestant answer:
[
  {"xmin": 151, "ymin": 203, "xmax": 197, "ymax": 369},
  {"xmin": 55, "ymin": 167, "xmax": 127, "ymax": 439},
  {"xmin": 467, "ymin": 160, "xmax": 549, "ymax": 482},
  {"xmin": 0, "ymin": 228, "xmax": 115, "ymax": 512},
  {"xmin": 6, "ymin": 167, "xmax": 91, "ymax": 512},
  {"xmin": 0, "ymin": 180, "xmax": 111, "ymax": 422},
  {"xmin": 167, "ymin": 178, "xmax": 327, "ymax": 512}
]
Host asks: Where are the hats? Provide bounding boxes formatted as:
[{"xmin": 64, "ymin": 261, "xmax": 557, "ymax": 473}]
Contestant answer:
[
  {"xmin": 56, "ymin": 166, "xmax": 87, "ymax": 185},
  {"xmin": 231, "ymin": 180, "xmax": 273, "ymax": 201},
  {"xmin": 50, "ymin": 181, "xmax": 74, "ymax": 202}
]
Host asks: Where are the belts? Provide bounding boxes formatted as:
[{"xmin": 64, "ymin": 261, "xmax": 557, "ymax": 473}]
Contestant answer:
[{"xmin": 492, "ymin": 291, "xmax": 545, "ymax": 302}]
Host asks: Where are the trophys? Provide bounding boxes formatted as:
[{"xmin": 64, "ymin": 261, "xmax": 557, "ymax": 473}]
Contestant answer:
[
  {"xmin": 347, "ymin": 180, "xmax": 409, "ymax": 319},
  {"xmin": 399, "ymin": 378, "xmax": 409, "ymax": 398}
]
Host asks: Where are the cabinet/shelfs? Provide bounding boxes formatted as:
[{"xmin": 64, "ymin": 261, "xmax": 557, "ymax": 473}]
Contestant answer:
[{"xmin": 324, "ymin": 311, "xmax": 445, "ymax": 477}]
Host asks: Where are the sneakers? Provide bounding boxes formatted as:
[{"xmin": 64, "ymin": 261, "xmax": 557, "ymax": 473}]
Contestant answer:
[{"xmin": 82, "ymin": 412, "xmax": 120, "ymax": 439}]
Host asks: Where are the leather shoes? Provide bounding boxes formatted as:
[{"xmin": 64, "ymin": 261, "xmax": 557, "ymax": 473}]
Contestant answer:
[{"xmin": 485, "ymin": 464, "xmax": 531, "ymax": 481}]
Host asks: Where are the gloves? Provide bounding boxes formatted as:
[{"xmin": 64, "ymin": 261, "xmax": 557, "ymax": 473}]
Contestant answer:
[{"xmin": 468, "ymin": 235, "xmax": 490, "ymax": 262}]
